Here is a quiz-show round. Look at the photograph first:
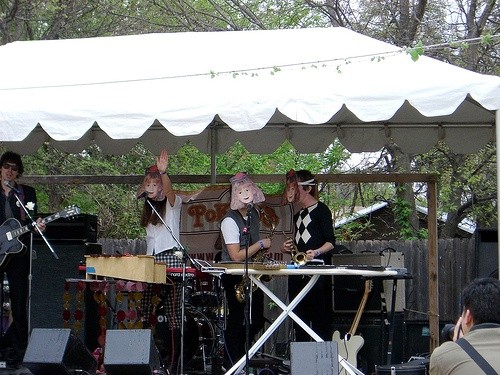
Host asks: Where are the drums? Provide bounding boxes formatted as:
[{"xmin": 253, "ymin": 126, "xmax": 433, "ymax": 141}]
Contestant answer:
[
  {"xmin": 151, "ymin": 301, "xmax": 219, "ymax": 368},
  {"xmin": 185, "ymin": 281, "xmax": 222, "ymax": 309}
]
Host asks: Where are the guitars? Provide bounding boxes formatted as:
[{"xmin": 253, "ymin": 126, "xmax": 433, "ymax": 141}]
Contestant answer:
[
  {"xmin": 0, "ymin": 204, "xmax": 80, "ymax": 266},
  {"xmin": 331, "ymin": 280, "xmax": 375, "ymax": 375}
]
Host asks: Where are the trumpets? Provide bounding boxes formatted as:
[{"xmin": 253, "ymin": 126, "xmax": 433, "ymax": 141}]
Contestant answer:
[{"xmin": 282, "ymin": 230, "xmax": 308, "ymax": 266}]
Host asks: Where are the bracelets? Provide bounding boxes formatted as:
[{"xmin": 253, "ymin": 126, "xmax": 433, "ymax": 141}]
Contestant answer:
[
  {"xmin": 159, "ymin": 172, "xmax": 167, "ymax": 175},
  {"xmin": 258, "ymin": 240, "xmax": 264, "ymax": 250},
  {"xmin": 316, "ymin": 249, "xmax": 320, "ymax": 256}
]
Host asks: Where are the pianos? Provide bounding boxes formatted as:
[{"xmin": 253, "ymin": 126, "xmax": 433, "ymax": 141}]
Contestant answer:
[{"xmin": 163, "ymin": 265, "xmax": 196, "ymax": 280}]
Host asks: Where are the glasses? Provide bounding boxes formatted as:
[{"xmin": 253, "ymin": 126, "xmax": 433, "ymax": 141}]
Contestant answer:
[{"xmin": 3, "ymin": 163, "xmax": 19, "ymax": 171}]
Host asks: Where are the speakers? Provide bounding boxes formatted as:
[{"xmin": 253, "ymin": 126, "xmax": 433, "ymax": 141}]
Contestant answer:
[
  {"xmin": 26, "ymin": 237, "xmax": 102, "ymax": 353},
  {"xmin": 332, "ymin": 252, "xmax": 405, "ymax": 314},
  {"xmin": 23, "ymin": 328, "xmax": 98, "ymax": 375},
  {"xmin": 104, "ymin": 330, "xmax": 169, "ymax": 375},
  {"xmin": 290, "ymin": 341, "xmax": 339, "ymax": 375},
  {"xmin": 474, "ymin": 228, "xmax": 498, "ymax": 279}
]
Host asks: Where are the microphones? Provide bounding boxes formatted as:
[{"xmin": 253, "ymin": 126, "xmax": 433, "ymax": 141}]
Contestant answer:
[
  {"xmin": 137, "ymin": 192, "xmax": 147, "ymax": 199},
  {"xmin": 4, "ymin": 181, "xmax": 20, "ymax": 193},
  {"xmin": 247, "ymin": 203, "xmax": 253, "ymax": 212}
]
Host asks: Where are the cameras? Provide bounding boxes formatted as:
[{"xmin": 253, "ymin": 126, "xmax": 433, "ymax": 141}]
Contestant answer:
[{"xmin": 442, "ymin": 324, "xmax": 456, "ymax": 342}]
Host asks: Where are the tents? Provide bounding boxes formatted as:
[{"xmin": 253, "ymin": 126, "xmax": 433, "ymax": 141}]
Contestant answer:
[{"xmin": 0, "ymin": 27, "xmax": 500, "ymax": 187}]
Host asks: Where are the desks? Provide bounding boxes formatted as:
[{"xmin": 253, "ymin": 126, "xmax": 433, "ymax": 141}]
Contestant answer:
[
  {"xmin": 65, "ymin": 278, "xmax": 166, "ymax": 371},
  {"xmin": 225, "ymin": 269, "xmax": 410, "ymax": 375}
]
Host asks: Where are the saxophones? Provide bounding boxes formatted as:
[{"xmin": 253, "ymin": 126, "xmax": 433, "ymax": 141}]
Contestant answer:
[{"xmin": 233, "ymin": 208, "xmax": 276, "ymax": 300}]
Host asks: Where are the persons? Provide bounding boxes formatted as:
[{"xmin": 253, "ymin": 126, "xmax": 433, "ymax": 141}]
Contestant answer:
[
  {"xmin": 0, "ymin": 151, "xmax": 46, "ymax": 369},
  {"xmin": 281, "ymin": 169, "xmax": 335, "ymax": 369},
  {"xmin": 141, "ymin": 149, "xmax": 186, "ymax": 374},
  {"xmin": 429, "ymin": 277, "xmax": 500, "ymax": 375},
  {"xmin": 220, "ymin": 172, "xmax": 272, "ymax": 375}
]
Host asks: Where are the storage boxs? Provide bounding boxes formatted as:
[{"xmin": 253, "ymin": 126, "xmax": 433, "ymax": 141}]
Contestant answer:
[
  {"xmin": 332, "ymin": 250, "xmax": 406, "ymax": 314},
  {"xmin": 29, "ymin": 243, "xmax": 103, "ymax": 350},
  {"xmin": 33, "ymin": 213, "xmax": 99, "ymax": 243}
]
